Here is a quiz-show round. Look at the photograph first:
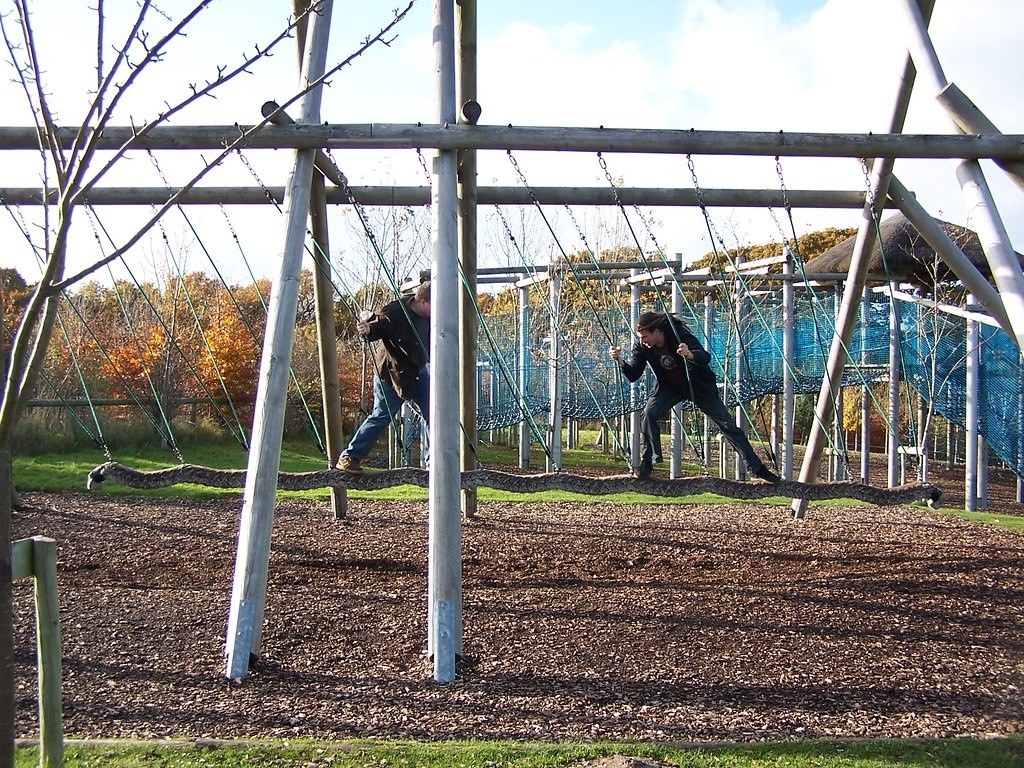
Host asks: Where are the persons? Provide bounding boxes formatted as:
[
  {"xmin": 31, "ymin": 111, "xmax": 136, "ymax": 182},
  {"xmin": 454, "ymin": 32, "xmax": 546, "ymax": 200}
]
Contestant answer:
[
  {"xmin": 609, "ymin": 312, "xmax": 780, "ymax": 483},
  {"xmin": 335, "ymin": 280, "xmax": 431, "ymax": 474}
]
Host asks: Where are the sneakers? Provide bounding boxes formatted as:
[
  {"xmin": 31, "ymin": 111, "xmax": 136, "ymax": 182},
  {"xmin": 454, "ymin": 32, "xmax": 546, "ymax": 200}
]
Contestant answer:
[
  {"xmin": 425, "ymin": 463, "xmax": 430, "ymax": 470},
  {"xmin": 335, "ymin": 456, "xmax": 363, "ymax": 474},
  {"xmin": 751, "ymin": 464, "xmax": 780, "ymax": 484},
  {"xmin": 633, "ymin": 458, "xmax": 653, "ymax": 479}
]
